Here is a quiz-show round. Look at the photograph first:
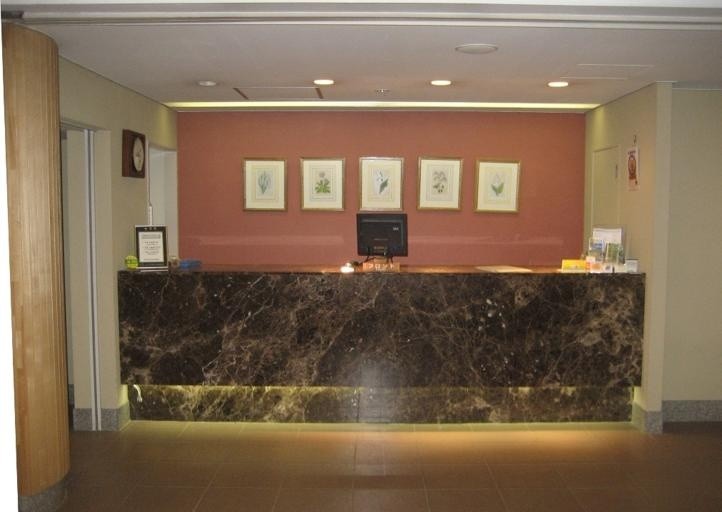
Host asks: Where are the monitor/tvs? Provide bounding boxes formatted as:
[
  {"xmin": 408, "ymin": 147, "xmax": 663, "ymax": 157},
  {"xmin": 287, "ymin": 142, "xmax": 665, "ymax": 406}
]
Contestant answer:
[{"xmin": 357, "ymin": 213, "xmax": 408, "ymax": 264}]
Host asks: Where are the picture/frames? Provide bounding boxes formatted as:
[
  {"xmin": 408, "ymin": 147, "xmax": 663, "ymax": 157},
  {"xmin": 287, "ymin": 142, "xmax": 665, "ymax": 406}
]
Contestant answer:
[{"xmin": 241, "ymin": 154, "xmax": 522, "ymax": 215}]
